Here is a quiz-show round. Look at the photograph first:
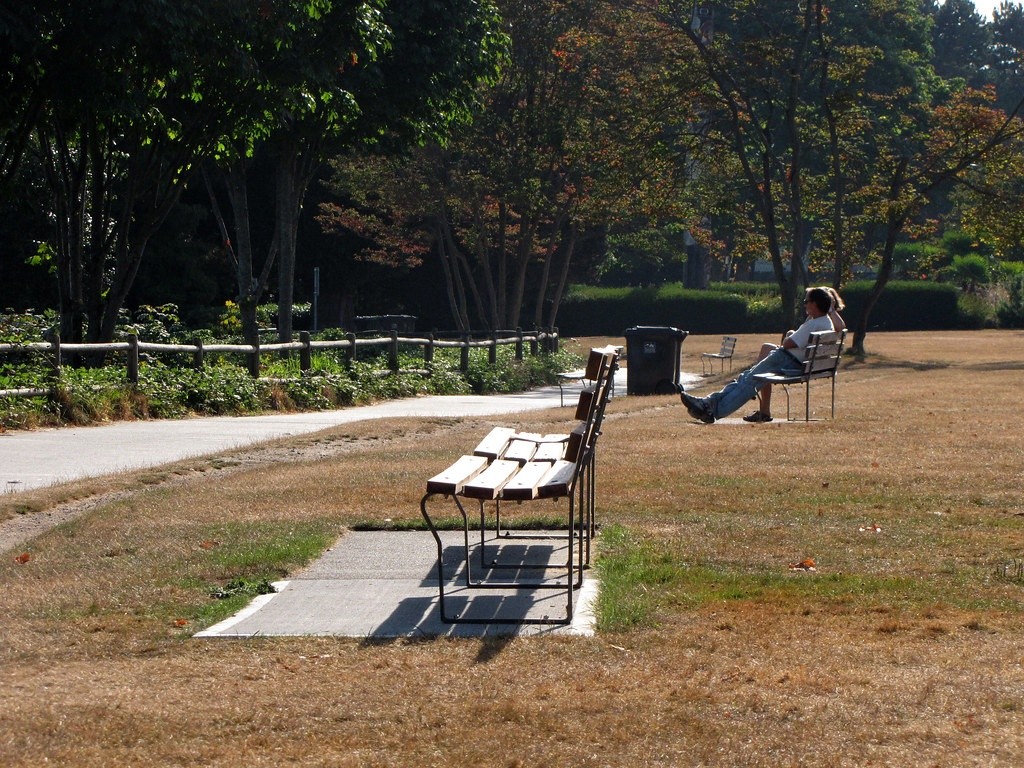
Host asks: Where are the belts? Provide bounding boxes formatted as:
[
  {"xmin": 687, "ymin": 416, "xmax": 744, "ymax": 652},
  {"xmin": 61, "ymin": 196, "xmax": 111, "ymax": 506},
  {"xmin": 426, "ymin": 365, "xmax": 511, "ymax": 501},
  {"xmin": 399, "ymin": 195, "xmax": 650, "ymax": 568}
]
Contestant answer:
[{"xmin": 779, "ymin": 347, "xmax": 803, "ymax": 366}]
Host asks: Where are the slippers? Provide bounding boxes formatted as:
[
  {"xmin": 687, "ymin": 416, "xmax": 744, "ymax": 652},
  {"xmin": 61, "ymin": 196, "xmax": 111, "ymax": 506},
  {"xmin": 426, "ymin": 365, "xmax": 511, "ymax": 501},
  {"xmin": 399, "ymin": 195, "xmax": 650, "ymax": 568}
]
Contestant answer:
[{"xmin": 743, "ymin": 410, "xmax": 773, "ymax": 422}]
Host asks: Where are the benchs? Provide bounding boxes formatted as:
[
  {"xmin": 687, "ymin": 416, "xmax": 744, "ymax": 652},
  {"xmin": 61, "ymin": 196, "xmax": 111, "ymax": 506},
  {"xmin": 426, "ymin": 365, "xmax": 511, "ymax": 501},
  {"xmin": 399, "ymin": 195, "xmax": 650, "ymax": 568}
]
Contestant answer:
[
  {"xmin": 753, "ymin": 328, "xmax": 849, "ymax": 424},
  {"xmin": 420, "ymin": 344, "xmax": 625, "ymax": 623},
  {"xmin": 556, "ymin": 345, "xmax": 624, "ymax": 407},
  {"xmin": 701, "ymin": 337, "xmax": 737, "ymax": 374}
]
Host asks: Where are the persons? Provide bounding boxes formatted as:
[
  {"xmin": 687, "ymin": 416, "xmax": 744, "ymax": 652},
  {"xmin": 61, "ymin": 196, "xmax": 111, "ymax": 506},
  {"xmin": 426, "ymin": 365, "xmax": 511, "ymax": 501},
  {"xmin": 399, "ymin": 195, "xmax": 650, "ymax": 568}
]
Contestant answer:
[
  {"xmin": 681, "ymin": 287, "xmax": 836, "ymax": 424},
  {"xmin": 743, "ymin": 287, "xmax": 846, "ymax": 422}
]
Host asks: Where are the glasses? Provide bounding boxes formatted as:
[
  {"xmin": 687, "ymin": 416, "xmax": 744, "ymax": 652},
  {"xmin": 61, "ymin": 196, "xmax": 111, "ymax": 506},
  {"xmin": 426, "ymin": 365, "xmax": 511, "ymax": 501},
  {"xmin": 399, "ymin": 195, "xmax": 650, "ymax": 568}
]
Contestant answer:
[{"xmin": 803, "ymin": 299, "xmax": 813, "ymax": 305}]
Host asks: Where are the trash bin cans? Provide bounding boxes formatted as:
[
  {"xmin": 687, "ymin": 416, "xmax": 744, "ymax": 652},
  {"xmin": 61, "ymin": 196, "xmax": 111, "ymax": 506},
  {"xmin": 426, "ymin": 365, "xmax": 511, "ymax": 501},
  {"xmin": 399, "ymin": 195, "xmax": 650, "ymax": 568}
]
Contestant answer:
[{"xmin": 621, "ymin": 325, "xmax": 690, "ymax": 396}]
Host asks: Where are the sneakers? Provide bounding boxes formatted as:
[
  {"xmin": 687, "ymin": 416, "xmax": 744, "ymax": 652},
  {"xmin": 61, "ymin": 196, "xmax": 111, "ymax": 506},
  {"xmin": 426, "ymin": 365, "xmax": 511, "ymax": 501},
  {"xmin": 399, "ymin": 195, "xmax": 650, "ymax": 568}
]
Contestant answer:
[
  {"xmin": 681, "ymin": 392, "xmax": 706, "ymax": 417},
  {"xmin": 687, "ymin": 408, "xmax": 714, "ymax": 423}
]
